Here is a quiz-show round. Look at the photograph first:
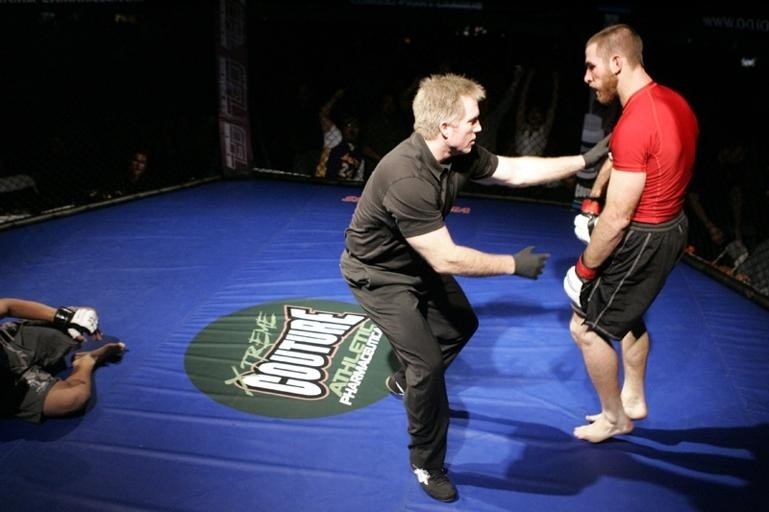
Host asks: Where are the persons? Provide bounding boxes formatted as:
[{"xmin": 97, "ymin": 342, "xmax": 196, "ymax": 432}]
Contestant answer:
[
  {"xmin": 360, "ymin": 95, "xmax": 397, "ymax": 180},
  {"xmin": 0, "ymin": 297, "xmax": 127, "ymax": 425},
  {"xmin": 338, "ymin": 72, "xmax": 613, "ymax": 502},
  {"xmin": 92, "ymin": 148, "xmax": 157, "ymax": 200},
  {"xmin": 686, "ymin": 138, "xmax": 749, "ymax": 247},
  {"xmin": 563, "ymin": 24, "xmax": 700, "ymax": 443},
  {"xmin": 476, "ymin": 65, "xmax": 525, "ymax": 153},
  {"xmin": 315, "ymin": 89, "xmax": 360, "ymax": 183},
  {"xmin": 514, "ymin": 67, "xmax": 561, "ymax": 156}
]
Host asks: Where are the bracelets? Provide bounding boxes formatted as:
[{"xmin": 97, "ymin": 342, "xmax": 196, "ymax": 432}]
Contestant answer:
[{"xmin": 53, "ymin": 306, "xmax": 74, "ymax": 332}]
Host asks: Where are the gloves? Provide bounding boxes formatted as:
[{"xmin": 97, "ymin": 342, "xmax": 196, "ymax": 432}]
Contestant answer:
[
  {"xmin": 50, "ymin": 304, "xmax": 104, "ymax": 343},
  {"xmin": 573, "ymin": 195, "xmax": 604, "ymax": 246},
  {"xmin": 580, "ymin": 130, "xmax": 613, "ymax": 171},
  {"xmin": 510, "ymin": 244, "xmax": 551, "ymax": 282},
  {"xmin": 563, "ymin": 252, "xmax": 601, "ymax": 310}
]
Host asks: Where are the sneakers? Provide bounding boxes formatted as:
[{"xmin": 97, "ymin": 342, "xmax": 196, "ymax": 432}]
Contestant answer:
[
  {"xmin": 384, "ymin": 372, "xmax": 409, "ymax": 402},
  {"xmin": 408, "ymin": 455, "xmax": 459, "ymax": 504}
]
